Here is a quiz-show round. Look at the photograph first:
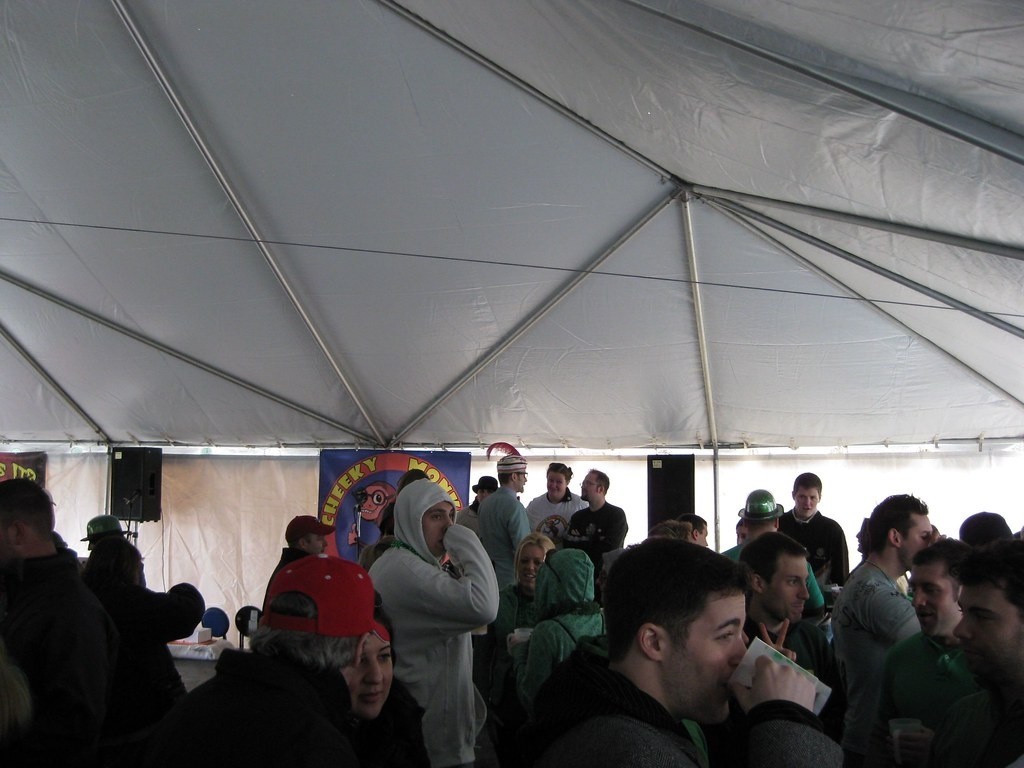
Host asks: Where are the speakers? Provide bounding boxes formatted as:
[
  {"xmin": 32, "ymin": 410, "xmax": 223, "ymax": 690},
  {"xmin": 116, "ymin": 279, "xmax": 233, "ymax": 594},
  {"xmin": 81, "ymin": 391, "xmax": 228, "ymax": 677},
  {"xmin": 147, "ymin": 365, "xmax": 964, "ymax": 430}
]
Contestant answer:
[
  {"xmin": 647, "ymin": 454, "xmax": 695, "ymax": 532},
  {"xmin": 110, "ymin": 447, "xmax": 163, "ymax": 523}
]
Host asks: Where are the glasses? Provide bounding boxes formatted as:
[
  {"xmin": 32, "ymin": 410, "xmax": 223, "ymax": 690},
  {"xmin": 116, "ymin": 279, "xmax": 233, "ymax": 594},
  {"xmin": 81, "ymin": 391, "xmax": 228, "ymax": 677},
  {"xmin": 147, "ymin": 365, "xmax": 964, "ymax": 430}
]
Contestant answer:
[
  {"xmin": 580, "ymin": 481, "xmax": 600, "ymax": 486},
  {"xmin": 545, "ymin": 549, "xmax": 562, "ymax": 583},
  {"xmin": 599, "ymin": 570, "xmax": 609, "ymax": 585}
]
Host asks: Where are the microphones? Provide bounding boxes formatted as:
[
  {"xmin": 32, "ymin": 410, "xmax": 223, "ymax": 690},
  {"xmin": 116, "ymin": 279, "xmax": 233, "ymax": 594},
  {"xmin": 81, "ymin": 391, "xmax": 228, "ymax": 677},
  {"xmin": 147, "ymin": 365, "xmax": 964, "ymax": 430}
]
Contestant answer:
[{"xmin": 357, "ymin": 505, "xmax": 361, "ymax": 537}]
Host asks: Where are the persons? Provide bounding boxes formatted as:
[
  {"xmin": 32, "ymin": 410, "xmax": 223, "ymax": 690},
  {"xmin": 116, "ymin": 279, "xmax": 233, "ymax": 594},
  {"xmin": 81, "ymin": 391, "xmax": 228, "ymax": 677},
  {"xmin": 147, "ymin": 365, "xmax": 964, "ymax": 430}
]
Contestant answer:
[{"xmin": 0, "ymin": 448, "xmax": 1024, "ymax": 767}]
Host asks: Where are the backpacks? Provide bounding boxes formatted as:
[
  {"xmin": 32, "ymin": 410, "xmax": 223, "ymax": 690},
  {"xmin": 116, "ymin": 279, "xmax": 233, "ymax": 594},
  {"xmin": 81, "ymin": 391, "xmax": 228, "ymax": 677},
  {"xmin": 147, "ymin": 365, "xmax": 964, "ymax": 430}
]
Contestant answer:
[{"xmin": 551, "ymin": 613, "xmax": 608, "ymax": 657}]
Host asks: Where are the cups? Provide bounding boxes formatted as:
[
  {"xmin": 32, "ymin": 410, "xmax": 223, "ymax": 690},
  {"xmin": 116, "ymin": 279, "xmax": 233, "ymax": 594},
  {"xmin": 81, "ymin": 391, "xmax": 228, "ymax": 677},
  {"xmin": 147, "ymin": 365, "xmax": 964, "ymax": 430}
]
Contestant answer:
[
  {"xmin": 725, "ymin": 637, "xmax": 832, "ymax": 715},
  {"xmin": 889, "ymin": 719, "xmax": 921, "ymax": 763},
  {"xmin": 507, "ymin": 628, "xmax": 533, "ymax": 656}
]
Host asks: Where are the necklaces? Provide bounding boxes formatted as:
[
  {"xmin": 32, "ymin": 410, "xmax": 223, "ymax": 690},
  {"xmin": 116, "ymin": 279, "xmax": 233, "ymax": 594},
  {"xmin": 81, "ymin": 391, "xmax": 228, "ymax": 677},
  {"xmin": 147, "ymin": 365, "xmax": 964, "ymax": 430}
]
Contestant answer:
[{"xmin": 864, "ymin": 559, "xmax": 898, "ymax": 591}]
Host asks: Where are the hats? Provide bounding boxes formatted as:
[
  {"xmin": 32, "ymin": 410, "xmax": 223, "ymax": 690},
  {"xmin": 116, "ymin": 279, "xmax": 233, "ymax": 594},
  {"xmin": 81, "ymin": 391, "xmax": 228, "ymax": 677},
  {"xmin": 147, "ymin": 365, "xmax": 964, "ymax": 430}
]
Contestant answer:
[
  {"xmin": 260, "ymin": 554, "xmax": 390, "ymax": 644},
  {"xmin": 473, "ymin": 476, "xmax": 498, "ymax": 494},
  {"xmin": 285, "ymin": 516, "xmax": 336, "ymax": 543},
  {"xmin": 738, "ymin": 490, "xmax": 784, "ymax": 520},
  {"xmin": 80, "ymin": 515, "xmax": 130, "ymax": 541},
  {"xmin": 486, "ymin": 442, "xmax": 528, "ymax": 474}
]
